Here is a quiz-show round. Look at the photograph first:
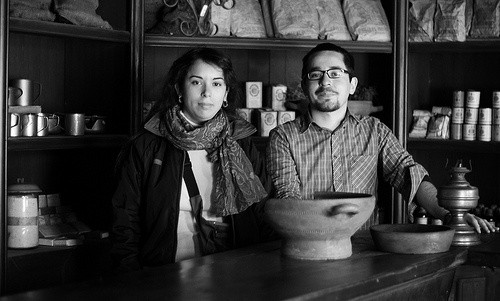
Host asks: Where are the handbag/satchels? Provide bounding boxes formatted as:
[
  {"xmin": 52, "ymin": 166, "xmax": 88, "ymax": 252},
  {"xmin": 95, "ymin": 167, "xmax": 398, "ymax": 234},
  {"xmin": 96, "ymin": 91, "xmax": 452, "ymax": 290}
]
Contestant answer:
[
  {"xmin": 10, "ymin": 0, "xmax": 55, "ymax": 22},
  {"xmin": 189, "ymin": 195, "xmax": 279, "ymax": 256}
]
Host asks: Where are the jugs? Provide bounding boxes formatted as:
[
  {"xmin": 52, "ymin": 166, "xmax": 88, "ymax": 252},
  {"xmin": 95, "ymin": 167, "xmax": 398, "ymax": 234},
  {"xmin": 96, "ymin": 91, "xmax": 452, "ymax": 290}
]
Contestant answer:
[
  {"xmin": 37, "ymin": 113, "xmax": 49, "ymax": 137},
  {"xmin": 8, "ymin": 86, "xmax": 23, "ymax": 106},
  {"xmin": 16, "ymin": 78, "xmax": 42, "ymax": 106},
  {"xmin": 10, "ymin": 112, "xmax": 21, "ymax": 137},
  {"xmin": 47, "ymin": 114, "xmax": 60, "ymax": 133},
  {"xmin": 22, "ymin": 114, "xmax": 37, "ymax": 137},
  {"xmin": 67, "ymin": 113, "xmax": 90, "ymax": 136}
]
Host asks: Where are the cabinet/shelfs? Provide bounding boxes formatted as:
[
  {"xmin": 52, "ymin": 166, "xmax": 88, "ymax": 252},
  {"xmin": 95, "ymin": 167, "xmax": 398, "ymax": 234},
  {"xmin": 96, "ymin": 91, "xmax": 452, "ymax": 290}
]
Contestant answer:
[{"xmin": 0, "ymin": 0, "xmax": 500, "ymax": 297}]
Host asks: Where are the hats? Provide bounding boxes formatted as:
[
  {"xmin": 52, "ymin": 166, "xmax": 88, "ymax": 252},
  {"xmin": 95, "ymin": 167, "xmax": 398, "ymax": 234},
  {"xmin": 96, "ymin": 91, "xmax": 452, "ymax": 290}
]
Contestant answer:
[{"xmin": 54, "ymin": 0, "xmax": 113, "ymax": 30}]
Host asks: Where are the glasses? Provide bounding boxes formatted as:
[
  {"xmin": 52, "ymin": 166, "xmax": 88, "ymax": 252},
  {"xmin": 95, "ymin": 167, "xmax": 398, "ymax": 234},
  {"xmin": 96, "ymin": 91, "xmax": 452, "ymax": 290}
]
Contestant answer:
[{"xmin": 304, "ymin": 68, "xmax": 353, "ymax": 81}]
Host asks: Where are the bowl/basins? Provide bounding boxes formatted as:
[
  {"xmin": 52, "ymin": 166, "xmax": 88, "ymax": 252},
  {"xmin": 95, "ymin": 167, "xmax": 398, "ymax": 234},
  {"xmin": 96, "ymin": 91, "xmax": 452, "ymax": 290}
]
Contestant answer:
[{"xmin": 369, "ymin": 224, "xmax": 455, "ymax": 254}]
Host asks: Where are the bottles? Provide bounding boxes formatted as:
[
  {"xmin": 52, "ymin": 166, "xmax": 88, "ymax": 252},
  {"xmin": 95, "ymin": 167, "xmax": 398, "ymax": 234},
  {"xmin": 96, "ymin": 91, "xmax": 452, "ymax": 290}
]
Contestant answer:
[
  {"xmin": 431, "ymin": 216, "xmax": 442, "ymax": 226},
  {"xmin": 417, "ymin": 206, "xmax": 429, "ymax": 225},
  {"xmin": 476, "ymin": 204, "xmax": 495, "ymax": 228}
]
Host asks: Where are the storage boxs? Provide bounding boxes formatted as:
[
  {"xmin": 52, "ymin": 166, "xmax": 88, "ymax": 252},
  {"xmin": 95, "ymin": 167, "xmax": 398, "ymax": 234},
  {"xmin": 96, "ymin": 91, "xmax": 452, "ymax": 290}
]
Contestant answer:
[{"xmin": 234, "ymin": 82, "xmax": 295, "ymax": 138}]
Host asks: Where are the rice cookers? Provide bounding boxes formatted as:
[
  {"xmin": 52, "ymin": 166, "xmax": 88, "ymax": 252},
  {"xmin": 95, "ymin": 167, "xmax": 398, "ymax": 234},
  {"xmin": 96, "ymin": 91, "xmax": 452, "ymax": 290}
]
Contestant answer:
[{"xmin": 84, "ymin": 116, "xmax": 106, "ymax": 132}]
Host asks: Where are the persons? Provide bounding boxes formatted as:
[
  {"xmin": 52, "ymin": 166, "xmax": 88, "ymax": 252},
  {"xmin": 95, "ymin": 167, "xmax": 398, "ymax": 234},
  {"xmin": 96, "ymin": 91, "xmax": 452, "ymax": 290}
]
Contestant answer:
[
  {"xmin": 110, "ymin": 46, "xmax": 274, "ymax": 278},
  {"xmin": 266, "ymin": 42, "xmax": 500, "ymax": 233}
]
